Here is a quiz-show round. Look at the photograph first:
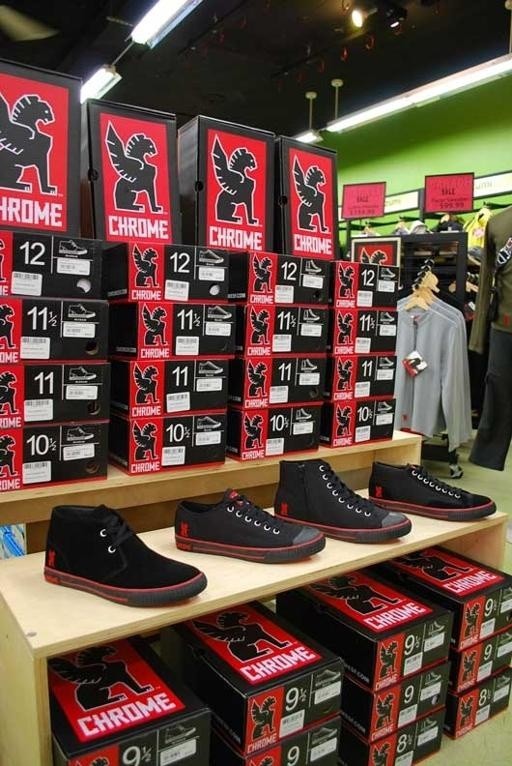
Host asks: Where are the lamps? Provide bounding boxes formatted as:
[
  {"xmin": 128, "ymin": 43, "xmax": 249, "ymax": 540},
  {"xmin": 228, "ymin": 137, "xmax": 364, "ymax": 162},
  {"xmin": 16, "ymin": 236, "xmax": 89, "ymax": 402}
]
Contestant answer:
[{"xmin": 351, "ymin": 6, "xmax": 379, "ymax": 28}]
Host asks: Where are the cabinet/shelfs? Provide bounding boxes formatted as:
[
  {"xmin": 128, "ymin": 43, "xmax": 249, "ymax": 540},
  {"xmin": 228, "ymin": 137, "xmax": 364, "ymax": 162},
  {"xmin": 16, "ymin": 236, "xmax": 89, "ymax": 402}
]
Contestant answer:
[
  {"xmin": 0, "ymin": 429, "xmax": 509, "ymax": 765},
  {"xmin": 0, "ymin": 430, "xmax": 423, "ymax": 555}
]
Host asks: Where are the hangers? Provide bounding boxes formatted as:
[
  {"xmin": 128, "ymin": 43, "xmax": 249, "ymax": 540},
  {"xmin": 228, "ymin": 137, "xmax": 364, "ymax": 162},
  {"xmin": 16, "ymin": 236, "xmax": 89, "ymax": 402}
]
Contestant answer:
[
  {"xmin": 403, "ymin": 255, "xmax": 444, "ymax": 318},
  {"xmin": 478, "ymin": 205, "xmax": 494, "ymax": 220},
  {"xmin": 361, "ymin": 222, "xmax": 375, "ymax": 234},
  {"xmin": 449, "ymin": 271, "xmax": 483, "ymax": 294},
  {"xmin": 437, "ymin": 211, "xmax": 459, "ymax": 224},
  {"xmin": 394, "ymin": 221, "xmax": 407, "ymax": 231}
]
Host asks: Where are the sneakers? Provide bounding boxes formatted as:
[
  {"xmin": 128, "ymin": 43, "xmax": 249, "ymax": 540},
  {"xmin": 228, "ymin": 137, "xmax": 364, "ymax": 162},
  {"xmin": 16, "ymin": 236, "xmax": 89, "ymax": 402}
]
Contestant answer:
[
  {"xmin": 368, "ymin": 461, "xmax": 497, "ymax": 521},
  {"xmin": 175, "ymin": 488, "xmax": 326, "ymax": 564},
  {"xmin": 274, "ymin": 459, "xmax": 412, "ymax": 543},
  {"xmin": 44, "ymin": 504, "xmax": 208, "ymax": 607}
]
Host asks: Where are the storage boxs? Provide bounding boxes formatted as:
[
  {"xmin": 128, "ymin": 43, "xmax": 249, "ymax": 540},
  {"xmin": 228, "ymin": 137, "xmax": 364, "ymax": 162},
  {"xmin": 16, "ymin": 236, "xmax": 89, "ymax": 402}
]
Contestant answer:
[
  {"xmin": 79, "ymin": 98, "xmax": 182, "ymax": 245},
  {"xmin": 342, "ymin": 659, "xmax": 451, "ymax": 743},
  {"xmin": 1, "ymin": 365, "xmax": 112, "ymax": 428},
  {"xmin": 322, "ymin": 400, "xmax": 396, "ymax": 449},
  {"xmin": 329, "ymin": 261, "xmax": 400, "ymax": 309},
  {"xmin": 450, "ymin": 629, "xmax": 511, "ymax": 693},
  {"xmin": 233, "ymin": 251, "xmax": 334, "ymax": 305},
  {"xmin": 325, "ymin": 357, "xmax": 396, "ymax": 400},
  {"xmin": 180, "ymin": 599, "xmax": 345, "ymax": 755},
  {"xmin": 114, "ymin": 366, "xmax": 231, "ymax": 417},
  {"xmin": 338, "ymin": 709, "xmax": 444, "ymax": 764},
  {"xmin": 0, "ymin": 298, "xmax": 111, "ymax": 361},
  {"xmin": 227, "ymin": 407, "xmax": 321, "ymax": 463},
  {"xmin": 113, "ymin": 422, "xmax": 230, "ymax": 476},
  {"xmin": 176, "ymin": 113, "xmax": 278, "ymax": 252},
  {"xmin": 444, "ymin": 668, "xmax": 510, "ymax": 741},
  {"xmin": 107, "ymin": 242, "xmax": 232, "ymax": 303},
  {"xmin": 330, "ymin": 312, "xmax": 398, "ymax": 354},
  {"xmin": 384, "ymin": 544, "xmax": 512, "ymax": 650},
  {"xmin": 113, "ymin": 304, "xmax": 238, "ymax": 362},
  {"xmin": 209, "ymin": 718, "xmax": 344, "ymax": 765},
  {"xmin": 0, "ymin": 231, "xmax": 104, "ymax": 300},
  {"xmin": 275, "ymin": 571, "xmax": 452, "ymax": 689},
  {"xmin": 231, "ymin": 356, "xmax": 327, "ymax": 409},
  {"xmin": 239, "ymin": 306, "xmax": 330, "ymax": 357},
  {"xmin": 277, "ymin": 134, "xmax": 341, "ymax": 260},
  {"xmin": 51, "ymin": 632, "xmax": 212, "ymax": 766},
  {"xmin": 0, "ymin": 55, "xmax": 84, "ymax": 239},
  {"xmin": 0, "ymin": 429, "xmax": 109, "ymax": 490}
]
